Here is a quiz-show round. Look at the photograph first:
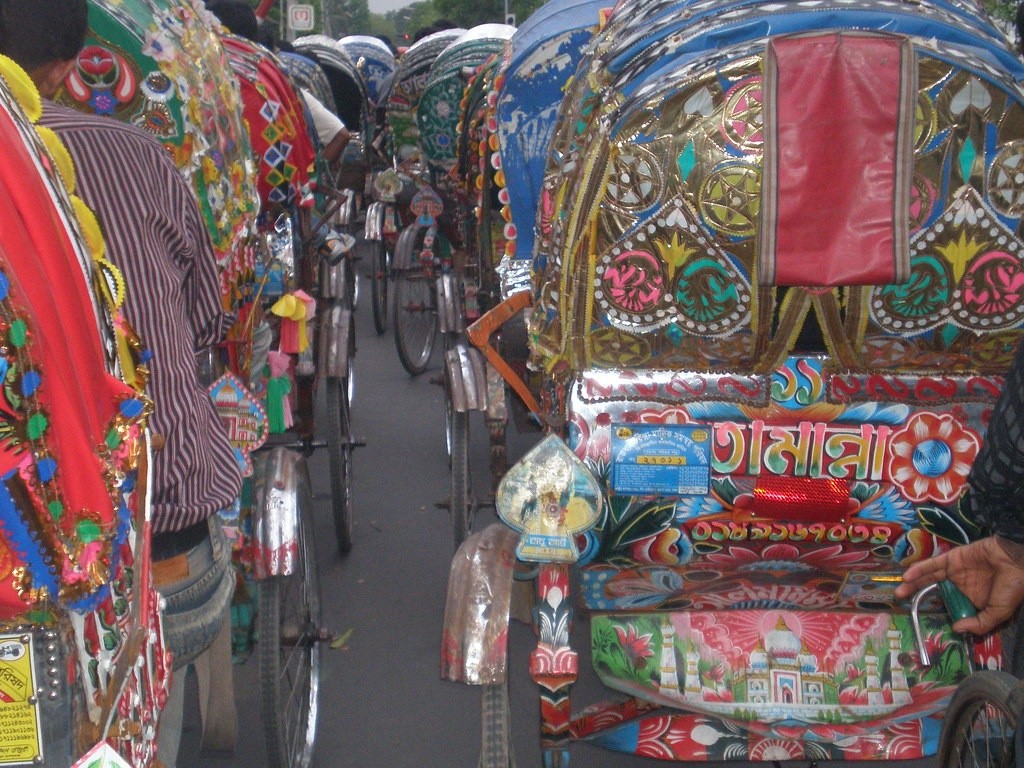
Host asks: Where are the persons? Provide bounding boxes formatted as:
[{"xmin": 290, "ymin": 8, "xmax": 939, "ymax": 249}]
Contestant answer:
[
  {"xmin": 1, "ymin": 0, "xmax": 245, "ymax": 672},
  {"xmin": 893, "ymin": 332, "xmax": 1024, "ymax": 630},
  {"xmin": 204, "ymin": 0, "xmax": 357, "ymax": 265},
  {"xmin": 259, "ymin": 17, "xmax": 461, "ymax": 75}
]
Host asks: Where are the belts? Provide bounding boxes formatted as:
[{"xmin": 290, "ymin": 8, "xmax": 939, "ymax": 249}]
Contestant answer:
[{"xmin": 152, "ymin": 520, "xmax": 208, "ymax": 562}]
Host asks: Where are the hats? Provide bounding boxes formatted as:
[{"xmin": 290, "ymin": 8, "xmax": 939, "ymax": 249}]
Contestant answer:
[{"xmin": 412, "ymin": 27, "xmax": 433, "ymax": 44}]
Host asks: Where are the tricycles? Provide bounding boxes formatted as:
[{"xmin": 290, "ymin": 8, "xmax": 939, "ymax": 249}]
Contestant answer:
[{"xmin": 0, "ymin": 2, "xmax": 1024, "ymax": 768}]
